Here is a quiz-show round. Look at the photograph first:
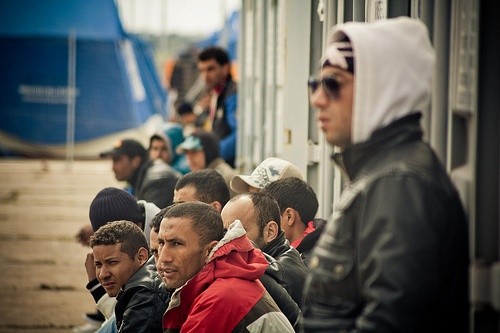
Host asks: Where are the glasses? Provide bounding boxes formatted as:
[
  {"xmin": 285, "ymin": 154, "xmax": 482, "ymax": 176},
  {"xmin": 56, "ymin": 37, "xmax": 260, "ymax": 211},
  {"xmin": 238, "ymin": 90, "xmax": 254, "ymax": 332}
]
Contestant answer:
[{"xmin": 307, "ymin": 75, "xmax": 354, "ymax": 101}]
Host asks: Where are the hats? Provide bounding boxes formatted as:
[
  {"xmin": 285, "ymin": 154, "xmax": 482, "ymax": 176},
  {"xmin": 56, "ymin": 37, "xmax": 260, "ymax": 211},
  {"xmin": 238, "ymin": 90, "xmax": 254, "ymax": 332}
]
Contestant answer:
[
  {"xmin": 89, "ymin": 187, "xmax": 143, "ymax": 232},
  {"xmin": 100, "ymin": 138, "xmax": 146, "ymax": 159},
  {"xmin": 320, "ymin": 34, "xmax": 355, "ymax": 76},
  {"xmin": 176, "ymin": 135, "xmax": 203, "ymax": 155},
  {"xmin": 230, "ymin": 157, "xmax": 305, "ymax": 195}
]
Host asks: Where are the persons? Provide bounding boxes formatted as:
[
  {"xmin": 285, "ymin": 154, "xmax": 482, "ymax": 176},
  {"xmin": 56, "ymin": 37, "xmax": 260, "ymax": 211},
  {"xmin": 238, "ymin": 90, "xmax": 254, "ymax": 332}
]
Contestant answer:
[
  {"xmin": 229, "ymin": 157, "xmax": 305, "ymax": 193},
  {"xmin": 75, "ymin": 138, "xmax": 184, "ymax": 246},
  {"xmin": 263, "ymin": 178, "xmax": 328, "ymax": 267},
  {"xmin": 89, "ymin": 220, "xmax": 176, "ymax": 333},
  {"xmin": 157, "ymin": 202, "xmax": 295, "ymax": 332},
  {"xmin": 150, "ymin": 203, "xmax": 302, "ymax": 333},
  {"xmin": 84, "ymin": 187, "xmax": 161, "ymax": 319},
  {"xmin": 299, "ymin": 15, "xmax": 471, "ymax": 333},
  {"xmin": 221, "ymin": 191, "xmax": 309, "ymax": 310},
  {"xmin": 146, "ymin": 40, "xmax": 240, "ymax": 197},
  {"xmin": 173, "ymin": 168, "xmax": 230, "ymax": 214}
]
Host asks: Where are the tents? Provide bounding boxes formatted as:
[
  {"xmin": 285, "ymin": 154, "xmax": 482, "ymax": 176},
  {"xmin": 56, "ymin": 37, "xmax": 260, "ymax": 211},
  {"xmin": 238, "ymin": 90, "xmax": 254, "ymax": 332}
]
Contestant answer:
[{"xmin": 0, "ymin": 0, "xmax": 170, "ymax": 159}]
